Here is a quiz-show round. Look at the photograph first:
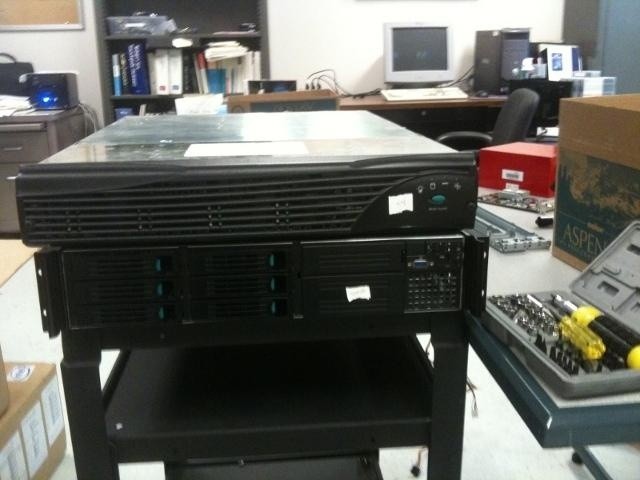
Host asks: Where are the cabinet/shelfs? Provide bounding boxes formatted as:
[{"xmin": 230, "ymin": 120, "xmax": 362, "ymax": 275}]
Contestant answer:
[{"xmin": 94, "ymin": 0, "xmax": 271, "ymax": 126}]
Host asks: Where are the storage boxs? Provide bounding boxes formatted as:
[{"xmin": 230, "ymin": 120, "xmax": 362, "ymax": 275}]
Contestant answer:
[
  {"xmin": 551, "ymin": 93, "xmax": 639, "ymax": 276},
  {"xmin": 480, "ymin": 141, "xmax": 556, "ymax": 199},
  {"xmin": 2, "ymin": 358, "xmax": 68, "ymax": 480}
]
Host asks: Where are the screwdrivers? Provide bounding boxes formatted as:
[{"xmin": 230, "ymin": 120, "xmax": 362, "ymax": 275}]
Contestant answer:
[{"xmin": 540, "ymin": 299, "xmax": 607, "ymax": 362}]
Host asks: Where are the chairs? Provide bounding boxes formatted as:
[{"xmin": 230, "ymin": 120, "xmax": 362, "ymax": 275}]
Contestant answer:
[{"xmin": 438, "ymin": 87, "xmax": 541, "ymax": 173}]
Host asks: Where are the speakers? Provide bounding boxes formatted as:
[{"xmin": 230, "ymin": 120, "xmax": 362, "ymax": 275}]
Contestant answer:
[{"xmin": 27, "ymin": 73, "xmax": 80, "ymax": 110}]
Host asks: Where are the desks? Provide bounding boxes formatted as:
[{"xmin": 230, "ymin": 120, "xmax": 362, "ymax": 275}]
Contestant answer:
[
  {"xmin": 336, "ymin": 90, "xmax": 510, "ymax": 146},
  {"xmin": 1, "ymin": 104, "xmax": 86, "ymax": 234}
]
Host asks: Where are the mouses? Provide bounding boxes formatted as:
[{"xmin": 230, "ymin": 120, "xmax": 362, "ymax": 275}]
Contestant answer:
[{"xmin": 475, "ymin": 90, "xmax": 488, "ymax": 98}]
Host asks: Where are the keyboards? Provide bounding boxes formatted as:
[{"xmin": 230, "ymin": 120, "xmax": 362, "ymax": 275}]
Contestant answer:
[{"xmin": 381, "ymin": 86, "xmax": 468, "ymax": 102}]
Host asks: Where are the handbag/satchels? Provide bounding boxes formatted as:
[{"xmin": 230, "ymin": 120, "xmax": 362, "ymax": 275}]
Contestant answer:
[{"xmin": 1, "ymin": 53, "xmax": 34, "ymax": 96}]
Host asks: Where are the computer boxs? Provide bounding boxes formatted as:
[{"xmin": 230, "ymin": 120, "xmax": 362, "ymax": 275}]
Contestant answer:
[{"xmin": 474, "ymin": 28, "xmax": 530, "ymax": 96}]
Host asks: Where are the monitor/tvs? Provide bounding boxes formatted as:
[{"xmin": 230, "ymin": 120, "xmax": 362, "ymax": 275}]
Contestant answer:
[{"xmin": 384, "ymin": 22, "xmax": 454, "ymax": 89}]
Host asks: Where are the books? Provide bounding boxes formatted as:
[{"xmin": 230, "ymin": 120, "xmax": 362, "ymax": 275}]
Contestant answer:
[
  {"xmin": 105, "ymin": 12, "xmax": 263, "ymax": 116},
  {"xmin": 0, "ymin": 93, "xmax": 36, "ymax": 120}
]
